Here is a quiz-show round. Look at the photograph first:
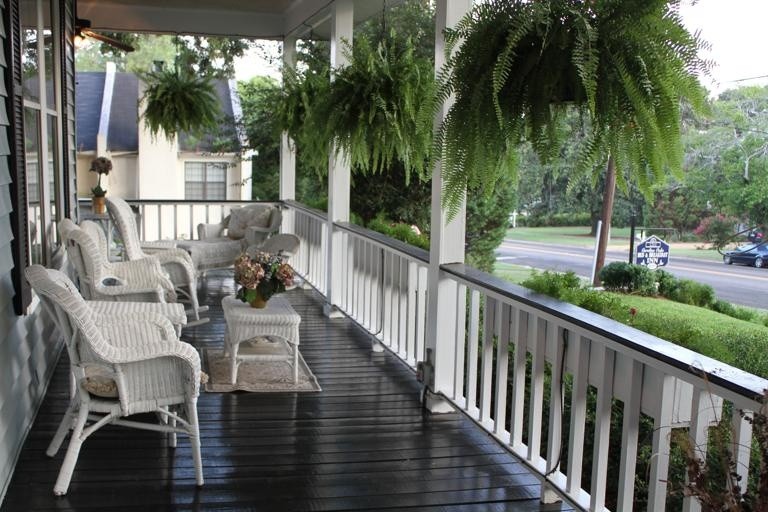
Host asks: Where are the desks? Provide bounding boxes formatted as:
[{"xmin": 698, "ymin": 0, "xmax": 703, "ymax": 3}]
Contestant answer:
[{"xmin": 221, "ymin": 293, "xmax": 299, "ymax": 384}]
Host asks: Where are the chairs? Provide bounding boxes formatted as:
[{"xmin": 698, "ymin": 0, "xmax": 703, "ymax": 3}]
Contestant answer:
[
  {"xmin": 119, "ymin": 202, "xmax": 281, "ymax": 274},
  {"xmin": 25, "ymin": 264, "xmax": 210, "ymax": 493},
  {"xmin": 58, "ymin": 219, "xmax": 167, "ymax": 305},
  {"xmin": 105, "ymin": 197, "xmax": 207, "ymax": 327}
]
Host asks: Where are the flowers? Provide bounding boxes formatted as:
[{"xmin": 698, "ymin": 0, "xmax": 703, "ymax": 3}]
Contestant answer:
[
  {"xmin": 87, "ymin": 157, "xmax": 111, "ymax": 198},
  {"xmin": 235, "ymin": 252, "xmax": 293, "ymax": 304}
]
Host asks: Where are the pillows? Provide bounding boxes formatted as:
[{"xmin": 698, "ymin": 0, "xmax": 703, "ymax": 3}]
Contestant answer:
[{"xmin": 228, "ymin": 206, "xmax": 272, "ymax": 238}]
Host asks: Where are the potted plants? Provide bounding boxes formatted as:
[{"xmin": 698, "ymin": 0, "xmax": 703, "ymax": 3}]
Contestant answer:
[{"xmin": 421, "ymin": 1, "xmax": 718, "ymax": 226}]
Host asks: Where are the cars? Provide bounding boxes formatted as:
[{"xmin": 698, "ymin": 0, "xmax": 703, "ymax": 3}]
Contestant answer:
[{"xmin": 723, "ymin": 243, "xmax": 768, "ymax": 268}]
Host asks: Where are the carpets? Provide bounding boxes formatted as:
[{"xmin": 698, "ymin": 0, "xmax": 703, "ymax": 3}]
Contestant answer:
[{"xmin": 200, "ymin": 346, "xmax": 322, "ymax": 392}]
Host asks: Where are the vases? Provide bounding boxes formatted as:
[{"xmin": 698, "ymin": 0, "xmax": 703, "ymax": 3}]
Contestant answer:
[{"xmin": 249, "ymin": 287, "xmax": 268, "ymax": 308}]
